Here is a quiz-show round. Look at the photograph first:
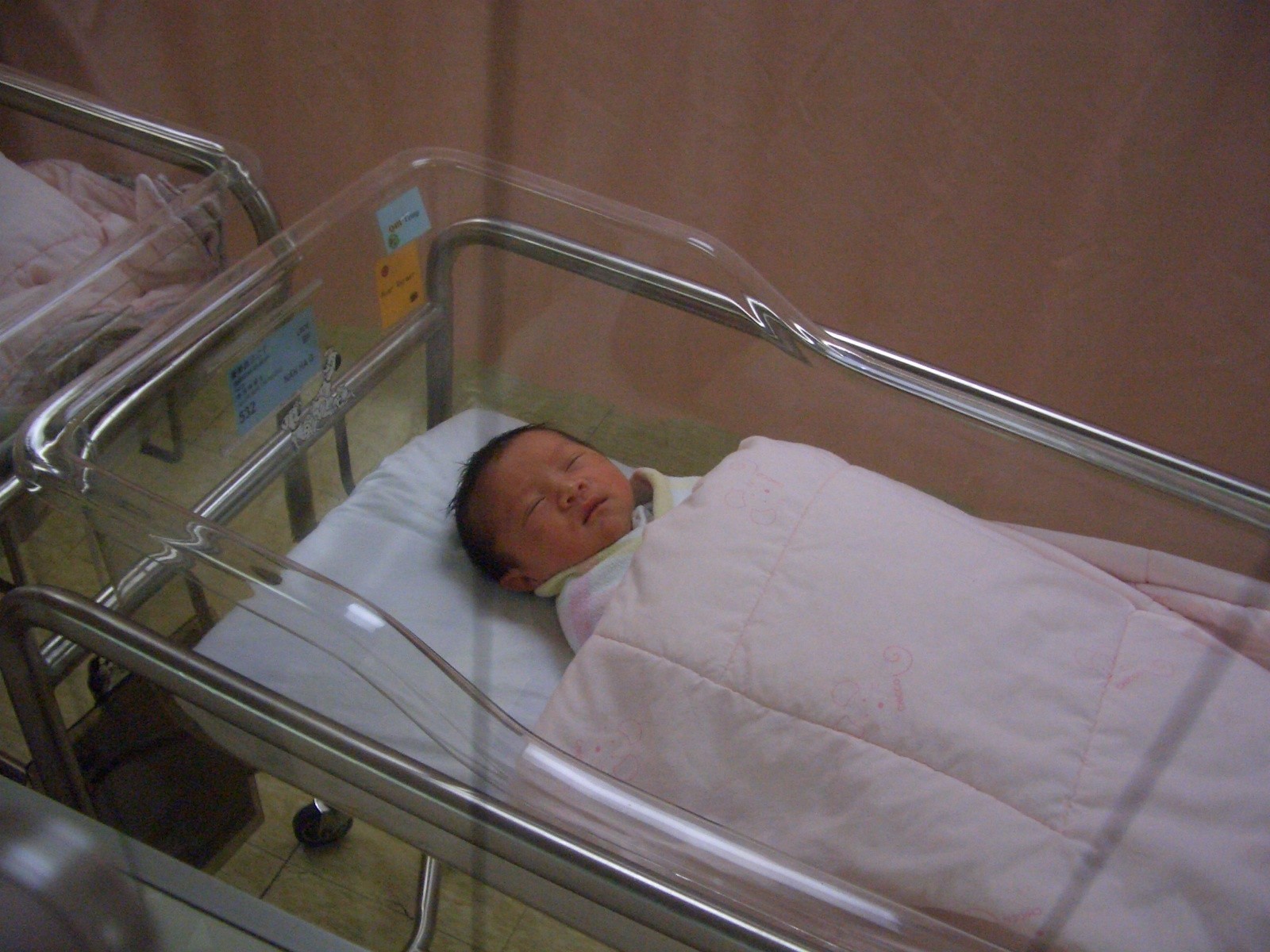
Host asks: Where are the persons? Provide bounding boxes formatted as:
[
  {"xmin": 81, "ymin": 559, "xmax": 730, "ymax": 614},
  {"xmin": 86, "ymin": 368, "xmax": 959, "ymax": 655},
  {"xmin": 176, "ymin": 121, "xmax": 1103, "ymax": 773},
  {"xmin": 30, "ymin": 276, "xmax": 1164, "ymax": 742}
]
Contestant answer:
[{"xmin": 447, "ymin": 422, "xmax": 704, "ymax": 658}]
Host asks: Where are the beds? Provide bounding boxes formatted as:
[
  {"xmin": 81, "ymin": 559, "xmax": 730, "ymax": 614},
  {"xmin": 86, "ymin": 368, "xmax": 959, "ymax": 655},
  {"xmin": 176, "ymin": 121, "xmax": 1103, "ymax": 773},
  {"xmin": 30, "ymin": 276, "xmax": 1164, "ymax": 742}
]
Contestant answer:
[{"xmin": 1, "ymin": 63, "xmax": 1270, "ymax": 952}]
